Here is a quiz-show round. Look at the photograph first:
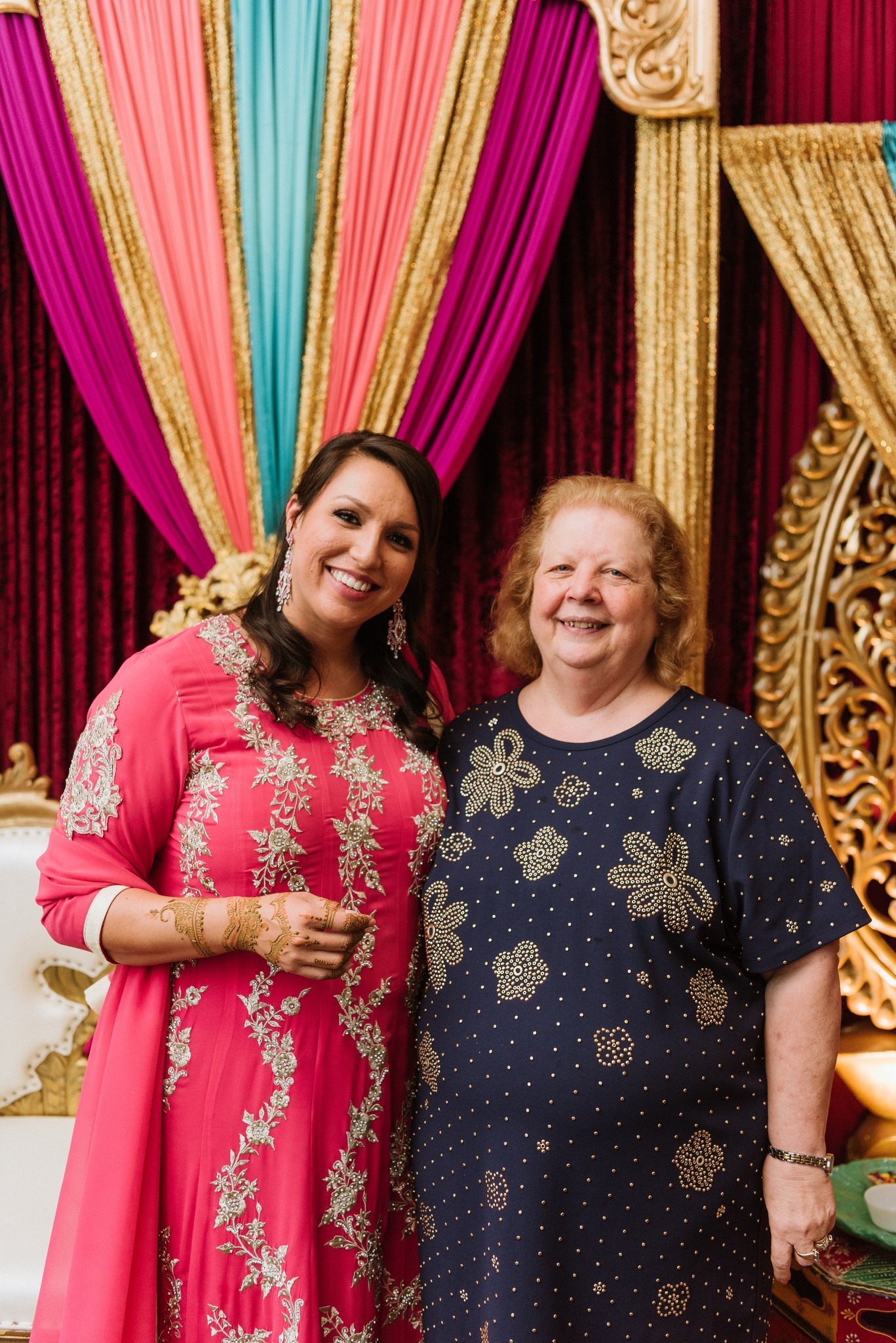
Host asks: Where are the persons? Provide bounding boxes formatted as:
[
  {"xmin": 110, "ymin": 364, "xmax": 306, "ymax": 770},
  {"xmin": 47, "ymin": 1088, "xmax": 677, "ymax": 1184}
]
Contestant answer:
[
  {"xmin": 444, "ymin": 476, "xmax": 874, "ymax": 1343},
  {"xmin": 27, "ymin": 433, "xmax": 465, "ymax": 1343}
]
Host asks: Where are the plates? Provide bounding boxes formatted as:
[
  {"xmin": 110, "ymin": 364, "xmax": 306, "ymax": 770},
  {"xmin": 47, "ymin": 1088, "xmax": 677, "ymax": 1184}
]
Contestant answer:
[{"xmin": 828, "ymin": 1156, "xmax": 896, "ymax": 1252}]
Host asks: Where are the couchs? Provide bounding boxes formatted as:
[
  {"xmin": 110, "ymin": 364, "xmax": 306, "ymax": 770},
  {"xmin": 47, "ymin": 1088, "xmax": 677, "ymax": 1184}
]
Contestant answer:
[{"xmin": 3, "ymin": 744, "xmax": 120, "ymax": 1338}]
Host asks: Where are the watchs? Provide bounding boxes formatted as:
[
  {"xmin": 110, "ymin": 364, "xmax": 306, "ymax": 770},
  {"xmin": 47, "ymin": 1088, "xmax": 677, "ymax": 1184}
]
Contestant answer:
[{"xmin": 768, "ymin": 1143, "xmax": 835, "ymax": 1174}]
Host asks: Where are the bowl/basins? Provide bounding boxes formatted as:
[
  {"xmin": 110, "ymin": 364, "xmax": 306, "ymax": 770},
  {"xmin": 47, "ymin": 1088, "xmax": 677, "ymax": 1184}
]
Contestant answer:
[{"xmin": 863, "ymin": 1183, "xmax": 895, "ymax": 1234}]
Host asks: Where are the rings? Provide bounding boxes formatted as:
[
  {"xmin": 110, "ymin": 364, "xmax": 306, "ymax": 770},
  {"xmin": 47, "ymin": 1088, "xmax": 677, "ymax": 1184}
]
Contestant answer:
[
  {"xmin": 792, "ymin": 1246, "xmax": 822, "ymax": 1261},
  {"xmin": 816, "ymin": 1233, "xmax": 833, "ymax": 1253}
]
